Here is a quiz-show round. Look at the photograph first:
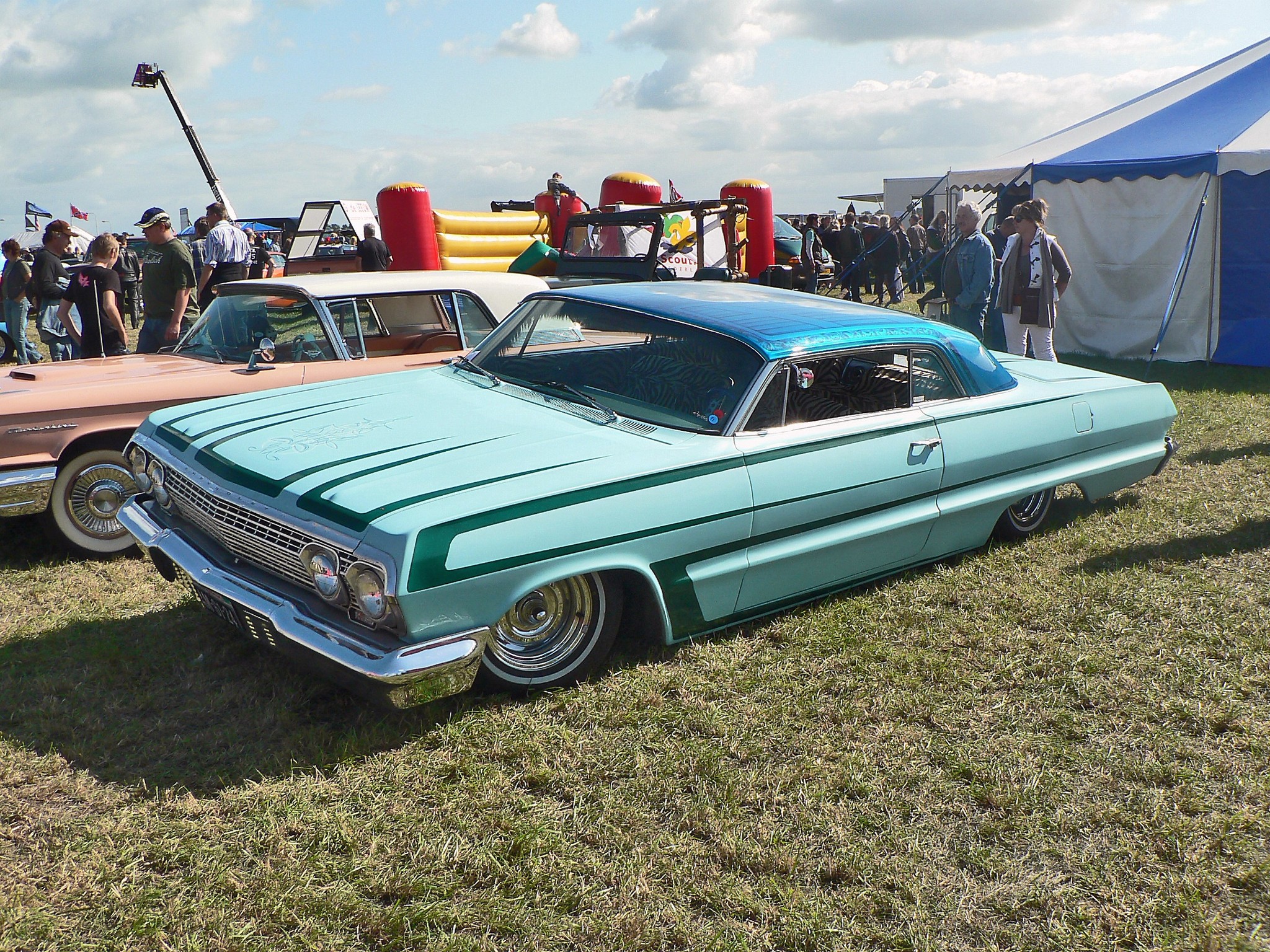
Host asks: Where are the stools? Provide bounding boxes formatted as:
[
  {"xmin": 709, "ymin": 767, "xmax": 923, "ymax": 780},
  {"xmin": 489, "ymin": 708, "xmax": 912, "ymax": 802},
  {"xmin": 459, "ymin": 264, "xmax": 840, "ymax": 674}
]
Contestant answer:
[{"xmin": 925, "ymin": 297, "xmax": 947, "ymax": 321}]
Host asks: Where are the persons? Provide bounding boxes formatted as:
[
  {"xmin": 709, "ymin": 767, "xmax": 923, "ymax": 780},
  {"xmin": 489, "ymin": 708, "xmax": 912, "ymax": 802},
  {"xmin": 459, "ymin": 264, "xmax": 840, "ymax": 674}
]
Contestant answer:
[
  {"xmin": 994, "ymin": 198, "xmax": 1071, "ymax": 362},
  {"xmin": 548, "ymin": 172, "xmax": 590, "ymax": 216},
  {"xmin": 941, "ymin": 201, "xmax": 996, "ymax": 345},
  {"xmin": 984, "ymin": 216, "xmax": 1030, "ymax": 355},
  {"xmin": 2, "ymin": 239, "xmax": 44, "ymax": 366},
  {"xmin": 355, "ymin": 223, "xmax": 393, "ymax": 271},
  {"xmin": 785, "ymin": 210, "xmax": 949, "ymax": 316},
  {"xmin": 20, "ymin": 202, "xmax": 358, "ymax": 362}
]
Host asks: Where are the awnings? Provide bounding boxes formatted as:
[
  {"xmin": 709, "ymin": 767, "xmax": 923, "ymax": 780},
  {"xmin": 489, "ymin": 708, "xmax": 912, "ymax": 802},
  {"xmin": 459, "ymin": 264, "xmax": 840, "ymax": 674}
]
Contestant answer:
[{"xmin": 837, "ymin": 193, "xmax": 883, "ymax": 210}]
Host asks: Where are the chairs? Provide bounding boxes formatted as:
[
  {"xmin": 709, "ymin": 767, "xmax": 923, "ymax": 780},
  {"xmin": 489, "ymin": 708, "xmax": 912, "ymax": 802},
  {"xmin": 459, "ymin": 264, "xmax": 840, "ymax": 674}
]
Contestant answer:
[
  {"xmin": 416, "ymin": 333, "xmax": 468, "ymax": 353},
  {"xmin": 751, "ymin": 390, "xmax": 859, "ymax": 428},
  {"xmin": 615, "ymin": 354, "xmax": 734, "ymax": 425}
]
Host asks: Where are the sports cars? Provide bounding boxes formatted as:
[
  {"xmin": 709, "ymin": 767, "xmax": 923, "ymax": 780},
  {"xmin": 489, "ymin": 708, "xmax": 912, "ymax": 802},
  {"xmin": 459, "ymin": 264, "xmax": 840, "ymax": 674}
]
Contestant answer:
[
  {"xmin": 0, "ymin": 270, "xmax": 676, "ymax": 561},
  {"xmin": 115, "ymin": 280, "xmax": 1177, "ymax": 708}
]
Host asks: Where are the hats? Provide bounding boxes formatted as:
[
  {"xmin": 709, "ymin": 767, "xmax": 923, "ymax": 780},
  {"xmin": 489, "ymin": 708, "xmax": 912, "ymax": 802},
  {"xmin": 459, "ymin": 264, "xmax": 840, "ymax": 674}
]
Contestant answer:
[
  {"xmin": 47, "ymin": 219, "xmax": 79, "ymax": 237},
  {"xmin": 22, "ymin": 247, "xmax": 31, "ymax": 252},
  {"xmin": 133, "ymin": 206, "xmax": 170, "ymax": 228}
]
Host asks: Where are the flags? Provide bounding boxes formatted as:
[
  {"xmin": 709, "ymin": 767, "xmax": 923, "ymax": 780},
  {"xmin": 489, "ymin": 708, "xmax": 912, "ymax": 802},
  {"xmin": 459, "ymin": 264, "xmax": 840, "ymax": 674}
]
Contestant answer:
[
  {"xmin": 669, "ymin": 179, "xmax": 684, "ymax": 202},
  {"xmin": 25, "ymin": 200, "xmax": 52, "ymax": 231},
  {"xmin": 71, "ymin": 203, "xmax": 88, "ymax": 221}
]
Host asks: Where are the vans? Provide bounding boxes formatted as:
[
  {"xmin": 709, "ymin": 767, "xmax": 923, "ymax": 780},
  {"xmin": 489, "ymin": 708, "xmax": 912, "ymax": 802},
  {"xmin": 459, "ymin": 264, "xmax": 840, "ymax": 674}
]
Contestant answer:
[{"xmin": 773, "ymin": 215, "xmax": 835, "ymax": 288}]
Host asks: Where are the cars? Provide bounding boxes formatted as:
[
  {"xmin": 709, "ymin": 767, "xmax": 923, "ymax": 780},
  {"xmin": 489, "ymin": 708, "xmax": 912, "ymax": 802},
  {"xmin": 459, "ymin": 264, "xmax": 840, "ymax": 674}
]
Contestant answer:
[{"xmin": 126, "ymin": 236, "xmax": 148, "ymax": 264}]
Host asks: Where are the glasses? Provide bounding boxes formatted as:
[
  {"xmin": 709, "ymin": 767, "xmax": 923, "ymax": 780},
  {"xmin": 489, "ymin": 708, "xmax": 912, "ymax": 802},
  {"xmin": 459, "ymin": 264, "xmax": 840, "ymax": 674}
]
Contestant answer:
[{"xmin": 1013, "ymin": 215, "xmax": 1026, "ymax": 223}]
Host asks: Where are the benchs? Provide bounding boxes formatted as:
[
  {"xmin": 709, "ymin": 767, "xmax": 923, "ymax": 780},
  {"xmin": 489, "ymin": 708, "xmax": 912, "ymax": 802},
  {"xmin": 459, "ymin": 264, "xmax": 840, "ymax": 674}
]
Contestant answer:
[{"xmin": 791, "ymin": 356, "xmax": 948, "ymax": 412}]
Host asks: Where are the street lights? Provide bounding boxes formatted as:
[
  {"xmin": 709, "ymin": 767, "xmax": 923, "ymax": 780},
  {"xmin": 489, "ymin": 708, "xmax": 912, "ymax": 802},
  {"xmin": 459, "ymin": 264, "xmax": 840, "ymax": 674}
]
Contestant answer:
[
  {"xmin": 101, "ymin": 220, "xmax": 112, "ymax": 233},
  {"xmin": 86, "ymin": 213, "xmax": 99, "ymax": 237}
]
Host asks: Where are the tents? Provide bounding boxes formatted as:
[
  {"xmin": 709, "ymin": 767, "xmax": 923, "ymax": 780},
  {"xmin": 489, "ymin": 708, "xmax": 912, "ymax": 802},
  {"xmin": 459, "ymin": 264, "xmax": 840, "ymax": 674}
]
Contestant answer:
[
  {"xmin": 0, "ymin": 226, "xmax": 96, "ymax": 258},
  {"xmin": 945, "ymin": 36, "xmax": 1270, "ymax": 364},
  {"xmin": 175, "ymin": 222, "xmax": 285, "ymax": 246}
]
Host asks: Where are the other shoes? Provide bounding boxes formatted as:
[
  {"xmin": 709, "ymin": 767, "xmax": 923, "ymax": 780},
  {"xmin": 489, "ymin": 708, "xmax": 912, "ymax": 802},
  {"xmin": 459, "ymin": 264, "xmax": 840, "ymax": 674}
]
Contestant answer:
[{"xmin": 917, "ymin": 299, "xmax": 925, "ymax": 315}]
